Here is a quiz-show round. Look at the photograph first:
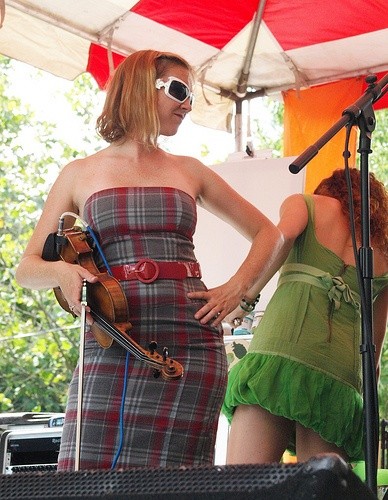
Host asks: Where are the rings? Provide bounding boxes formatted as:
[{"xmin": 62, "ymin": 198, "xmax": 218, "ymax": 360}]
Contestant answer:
[{"xmin": 69, "ymin": 305, "xmax": 75, "ymax": 312}]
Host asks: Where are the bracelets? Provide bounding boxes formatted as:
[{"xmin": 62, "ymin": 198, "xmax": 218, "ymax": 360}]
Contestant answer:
[{"xmin": 239, "ymin": 295, "xmax": 261, "ymax": 312}]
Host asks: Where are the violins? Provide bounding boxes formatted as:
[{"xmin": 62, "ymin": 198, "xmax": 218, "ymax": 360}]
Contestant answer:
[{"xmin": 41, "ymin": 226, "xmax": 184, "ymax": 381}]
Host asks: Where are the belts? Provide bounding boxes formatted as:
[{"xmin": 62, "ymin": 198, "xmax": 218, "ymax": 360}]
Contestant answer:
[{"xmin": 98, "ymin": 259, "xmax": 202, "ymax": 283}]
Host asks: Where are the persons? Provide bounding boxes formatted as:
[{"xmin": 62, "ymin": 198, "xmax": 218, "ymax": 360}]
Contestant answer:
[
  {"xmin": 13, "ymin": 49, "xmax": 284, "ymax": 475},
  {"xmin": 219, "ymin": 169, "xmax": 388, "ymax": 466}
]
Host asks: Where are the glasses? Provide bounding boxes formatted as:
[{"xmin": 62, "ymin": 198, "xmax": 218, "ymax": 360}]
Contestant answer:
[{"xmin": 155, "ymin": 76, "xmax": 195, "ymax": 106}]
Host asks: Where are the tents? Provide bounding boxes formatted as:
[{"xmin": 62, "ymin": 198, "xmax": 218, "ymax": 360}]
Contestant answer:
[{"xmin": 3, "ymin": 0, "xmax": 387, "ymax": 157}]
